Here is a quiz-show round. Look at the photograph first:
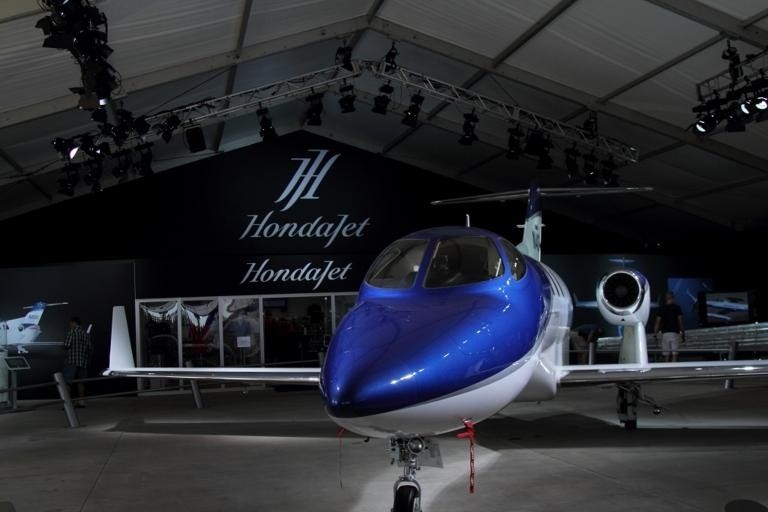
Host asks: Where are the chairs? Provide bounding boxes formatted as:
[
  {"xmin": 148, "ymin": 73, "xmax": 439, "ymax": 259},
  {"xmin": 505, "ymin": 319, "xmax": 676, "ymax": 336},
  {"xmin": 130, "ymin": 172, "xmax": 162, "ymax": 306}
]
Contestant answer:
[{"xmin": 428, "ymin": 240, "xmax": 471, "ymax": 285}]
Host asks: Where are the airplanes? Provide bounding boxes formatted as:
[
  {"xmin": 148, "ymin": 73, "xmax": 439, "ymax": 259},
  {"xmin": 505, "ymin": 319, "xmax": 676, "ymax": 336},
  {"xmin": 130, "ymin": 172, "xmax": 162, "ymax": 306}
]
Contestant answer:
[
  {"xmin": 103, "ymin": 183, "xmax": 768, "ymax": 512},
  {"xmin": 0, "ymin": 301, "xmax": 92, "ymax": 355}
]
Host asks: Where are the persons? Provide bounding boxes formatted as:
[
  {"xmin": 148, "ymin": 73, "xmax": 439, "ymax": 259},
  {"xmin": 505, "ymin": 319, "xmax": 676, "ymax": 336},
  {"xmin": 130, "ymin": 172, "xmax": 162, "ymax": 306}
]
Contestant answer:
[
  {"xmin": 653, "ymin": 291, "xmax": 685, "ymax": 362},
  {"xmin": 579, "ymin": 324, "xmax": 604, "ymax": 363},
  {"xmin": 146, "ymin": 305, "xmax": 289, "ymax": 368},
  {"xmin": 60, "ymin": 316, "xmax": 96, "ymax": 410}
]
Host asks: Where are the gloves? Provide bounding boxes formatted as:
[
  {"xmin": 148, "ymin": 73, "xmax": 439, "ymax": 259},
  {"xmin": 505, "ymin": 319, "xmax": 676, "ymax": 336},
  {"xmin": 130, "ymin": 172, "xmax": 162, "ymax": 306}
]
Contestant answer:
[
  {"xmin": 681, "ymin": 331, "xmax": 686, "ymax": 344},
  {"xmin": 652, "ymin": 334, "xmax": 659, "ymax": 338}
]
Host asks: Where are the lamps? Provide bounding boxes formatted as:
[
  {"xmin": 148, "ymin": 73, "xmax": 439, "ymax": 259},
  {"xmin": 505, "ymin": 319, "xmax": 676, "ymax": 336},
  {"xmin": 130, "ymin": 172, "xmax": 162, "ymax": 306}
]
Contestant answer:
[
  {"xmin": 307, "ymin": 38, "xmax": 424, "ymax": 129},
  {"xmin": 254, "ymin": 102, "xmax": 279, "ymax": 142},
  {"xmin": 457, "ymin": 107, "xmax": 480, "ymax": 147}
]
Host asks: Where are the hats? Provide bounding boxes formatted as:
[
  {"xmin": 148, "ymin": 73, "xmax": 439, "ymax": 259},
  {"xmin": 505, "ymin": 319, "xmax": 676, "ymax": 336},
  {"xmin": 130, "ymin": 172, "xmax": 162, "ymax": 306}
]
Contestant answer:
[{"xmin": 665, "ymin": 292, "xmax": 674, "ymax": 298}]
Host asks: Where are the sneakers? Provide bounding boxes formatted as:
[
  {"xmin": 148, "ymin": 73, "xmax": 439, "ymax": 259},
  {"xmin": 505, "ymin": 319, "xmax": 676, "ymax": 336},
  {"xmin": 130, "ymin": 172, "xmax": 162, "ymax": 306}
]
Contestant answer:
[{"xmin": 61, "ymin": 403, "xmax": 86, "ymax": 411}]
